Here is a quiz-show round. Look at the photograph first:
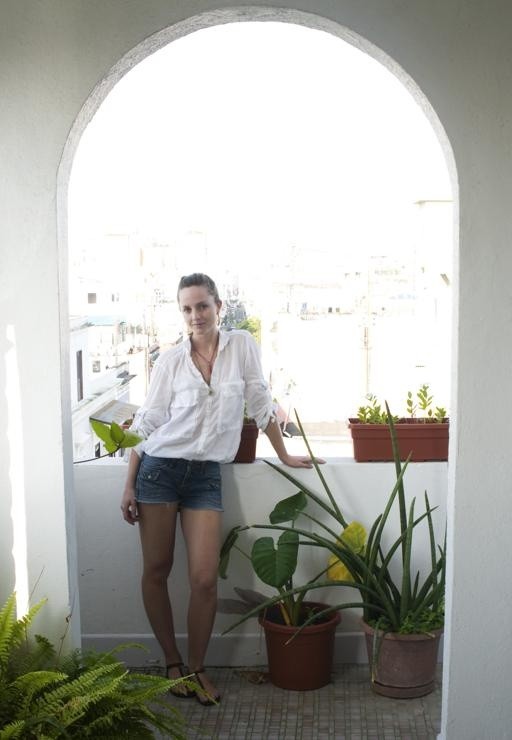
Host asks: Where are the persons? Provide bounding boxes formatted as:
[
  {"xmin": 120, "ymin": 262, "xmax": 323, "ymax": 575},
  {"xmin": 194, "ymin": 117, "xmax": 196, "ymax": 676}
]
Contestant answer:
[{"xmin": 122, "ymin": 275, "xmax": 326, "ymax": 708}]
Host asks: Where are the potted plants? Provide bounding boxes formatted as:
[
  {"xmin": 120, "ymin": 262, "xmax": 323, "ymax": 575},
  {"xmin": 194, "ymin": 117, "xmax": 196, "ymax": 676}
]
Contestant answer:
[
  {"xmin": 347, "ymin": 385, "xmax": 450, "ymax": 462},
  {"xmin": 218, "ymin": 400, "xmax": 447, "ymax": 700},
  {"xmin": 225, "ymin": 322, "xmax": 260, "ymax": 463},
  {"xmin": 218, "ymin": 492, "xmax": 368, "ymax": 691}
]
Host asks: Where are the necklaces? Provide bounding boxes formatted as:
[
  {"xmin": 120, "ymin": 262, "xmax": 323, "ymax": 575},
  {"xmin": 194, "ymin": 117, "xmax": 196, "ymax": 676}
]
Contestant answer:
[{"xmin": 191, "ymin": 335, "xmax": 219, "ymax": 395}]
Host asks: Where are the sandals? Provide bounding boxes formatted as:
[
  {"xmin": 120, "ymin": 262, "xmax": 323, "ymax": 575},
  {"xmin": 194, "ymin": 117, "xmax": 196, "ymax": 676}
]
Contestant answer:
[
  {"xmin": 165, "ymin": 663, "xmax": 196, "ymax": 698},
  {"xmin": 187, "ymin": 669, "xmax": 220, "ymax": 706}
]
